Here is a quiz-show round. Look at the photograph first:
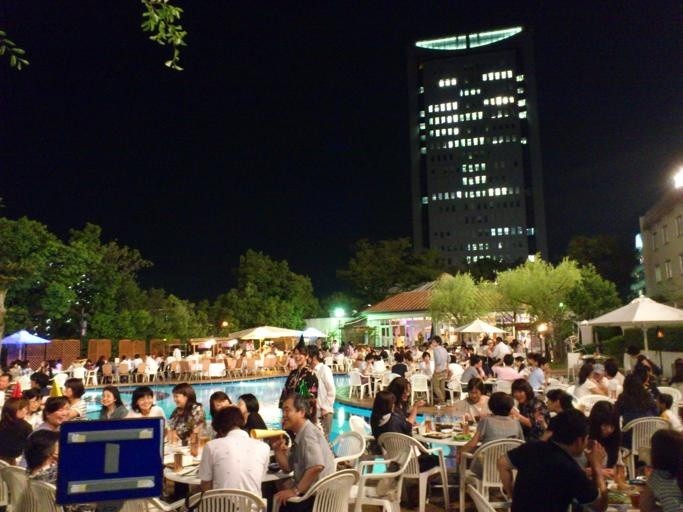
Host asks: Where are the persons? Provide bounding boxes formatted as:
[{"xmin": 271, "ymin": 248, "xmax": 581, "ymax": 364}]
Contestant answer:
[
  {"xmin": 498, "ymin": 410, "xmax": 607, "ymax": 512},
  {"xmin": 339, "ymin": 336, "xmax": 682, "ymax": 506},
  {"xmin": 638, "ymin": 429, "xmax": 683, "ymax": 512},
  {"xmin": 198, "ymin": 405, "xmax": 271, "ymax": 512},
  {"xmin": 273, "ymin": 394, "xmax": 335, "ymax": 512},
  {"xmin": 1, "ymin": 335, "xmax": 338, "ymax": 485}
]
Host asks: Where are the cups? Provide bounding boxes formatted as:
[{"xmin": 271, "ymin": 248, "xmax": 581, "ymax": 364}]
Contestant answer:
[
  {"xmin": 173, "ymin": 451, "xmax": 183, "ymax": 473},
  {"xmin": 191, "ymin": 429, "xmax": 199, "ymax": 458},
  {"xmin": 425, "ymin": 415, "xmax": 433, "ymax": 432}
]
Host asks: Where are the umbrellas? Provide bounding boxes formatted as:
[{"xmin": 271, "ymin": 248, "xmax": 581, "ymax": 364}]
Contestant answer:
[
  {"xmin": 0, "ymin": 328, "xmax": 51, "ymax": 359},
  {"xmin": 228, "ymin": 326, "xmax": 303, "ymax": 340},
  {"xmin": 579, "ymin": 295, "xmax": 682, "ymax": 354},
  {"xmin": 302, "ymin": 327, "xmax": 330, "ymax": 338},
  {"xmin": 452, "ymin": 317, "xmax": 509, "ymax": 333}
]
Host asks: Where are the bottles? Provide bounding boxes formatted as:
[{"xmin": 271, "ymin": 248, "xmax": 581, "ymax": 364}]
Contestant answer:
[{"xmin": 616, "ymin": 448, "xmax": 625, "ymax": 482}]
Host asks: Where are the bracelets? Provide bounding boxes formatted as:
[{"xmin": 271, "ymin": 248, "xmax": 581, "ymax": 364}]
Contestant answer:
[{"xmin": 293, "ymin": 485, "xmax": 300, "ymax": 496}]
